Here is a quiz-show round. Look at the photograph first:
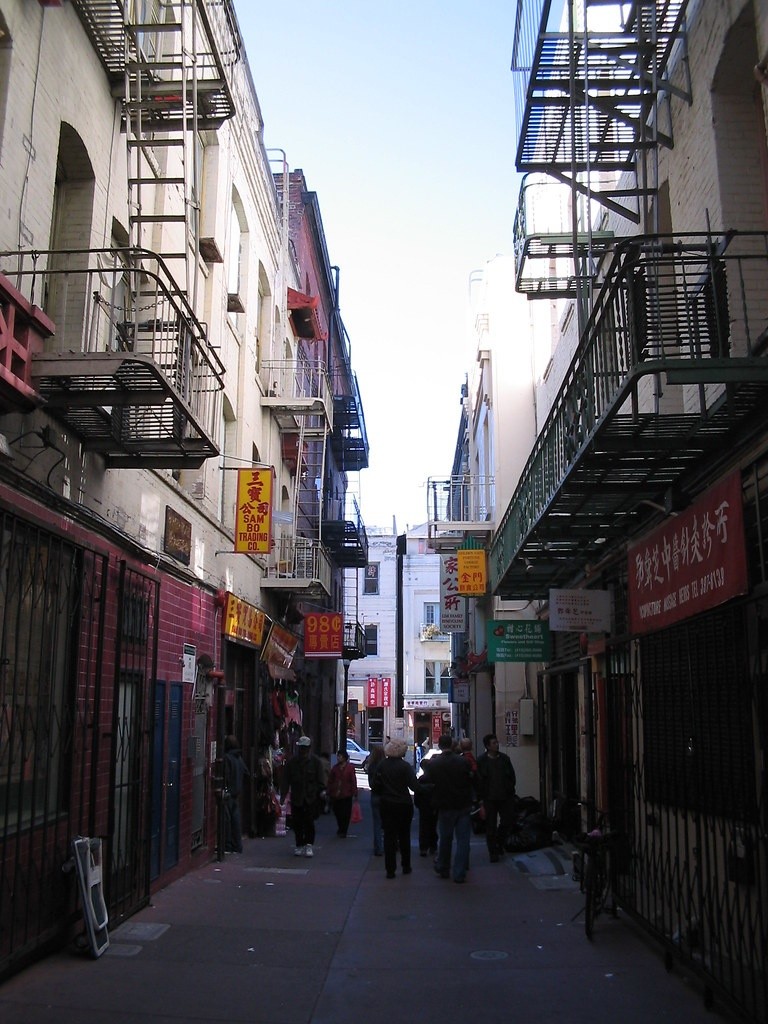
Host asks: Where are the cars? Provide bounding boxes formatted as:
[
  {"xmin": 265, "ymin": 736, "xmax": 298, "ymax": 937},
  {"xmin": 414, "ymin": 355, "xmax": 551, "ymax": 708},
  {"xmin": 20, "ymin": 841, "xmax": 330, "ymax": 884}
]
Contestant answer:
[{"xmin": 346, "ymin": 738, "xmax": 372, "ymax": 772}]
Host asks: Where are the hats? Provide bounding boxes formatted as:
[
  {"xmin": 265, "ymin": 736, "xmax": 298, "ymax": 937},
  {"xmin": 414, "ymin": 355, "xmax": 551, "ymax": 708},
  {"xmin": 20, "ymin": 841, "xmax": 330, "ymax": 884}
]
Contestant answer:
[{"xmin": 299, "ymin": 736, "xmax": 311, "ymax": 746}]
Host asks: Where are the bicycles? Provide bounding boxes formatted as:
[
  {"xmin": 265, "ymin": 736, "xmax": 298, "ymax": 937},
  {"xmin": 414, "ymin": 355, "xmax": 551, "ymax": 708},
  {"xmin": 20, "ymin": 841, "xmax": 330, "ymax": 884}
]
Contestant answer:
[{"xmin": 562, "ymin": 798, "xmax": 633, "ymax": 939}]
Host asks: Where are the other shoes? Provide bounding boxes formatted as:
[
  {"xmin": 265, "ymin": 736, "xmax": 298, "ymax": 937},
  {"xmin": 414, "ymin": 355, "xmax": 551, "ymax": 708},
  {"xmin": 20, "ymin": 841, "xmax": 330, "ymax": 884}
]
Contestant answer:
[
  {"xmin": 403, "ymin": 866, "xmax": 411, "ymax": 873},
  {"xmin": 386, "ymin": 872, "xmax": 396, "ymax": 879},
  {"xmin": 420, "ymin": 850, "xmax": 427, "ymax": 857},
  {"xmin": 432, "ymin": 850, "xmax": 438, "ymax": 856}
]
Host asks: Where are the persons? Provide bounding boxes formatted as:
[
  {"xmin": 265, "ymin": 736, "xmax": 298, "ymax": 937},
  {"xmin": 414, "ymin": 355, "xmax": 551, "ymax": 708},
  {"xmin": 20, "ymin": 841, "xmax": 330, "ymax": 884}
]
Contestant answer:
[
  {"xmin": 326, "ymin": 749, "xmax": 358, "ymax": 838},
  {"xmin": 222, "ymin": 735, "xmax": 250, "ymax": 854},
  {"xmin": 290, "ymin": 736, "xmax": 325, "ymax": 858},
  {"xmin": 367, "ymin": 733, "xmax": 516, "ymax": 883}
]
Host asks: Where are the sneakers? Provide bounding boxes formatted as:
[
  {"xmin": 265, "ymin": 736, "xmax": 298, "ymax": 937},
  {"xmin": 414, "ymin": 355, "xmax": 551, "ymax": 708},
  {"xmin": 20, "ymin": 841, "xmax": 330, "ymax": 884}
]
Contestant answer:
[
  {"xmin": 295, "ymin": 846, "xmax": 305, "ymax": 855},
  {"xmin": 304, "ymin": 844, "xmax": 313, "ymax": 857}
]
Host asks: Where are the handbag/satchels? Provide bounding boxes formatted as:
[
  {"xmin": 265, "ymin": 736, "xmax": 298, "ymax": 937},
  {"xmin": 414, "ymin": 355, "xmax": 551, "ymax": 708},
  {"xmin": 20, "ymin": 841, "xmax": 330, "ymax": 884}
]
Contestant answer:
[{"xmin": 351, "ymin": 800, "xmax": 363, "ymax": 823}]
